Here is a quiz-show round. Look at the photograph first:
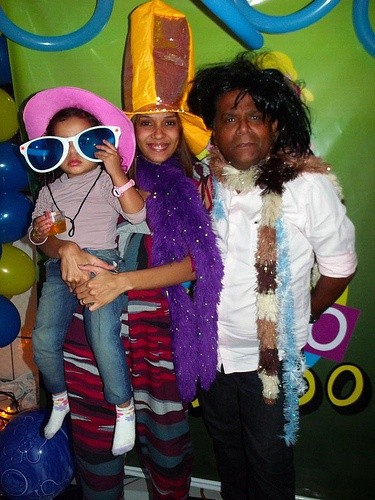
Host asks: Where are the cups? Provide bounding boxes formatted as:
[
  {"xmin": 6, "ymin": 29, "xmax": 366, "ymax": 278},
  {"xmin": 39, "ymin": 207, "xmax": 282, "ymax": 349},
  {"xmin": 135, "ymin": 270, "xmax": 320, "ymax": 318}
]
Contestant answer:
[{"xmin": 48, "ymin": 210, "xmax": 67, "ymax": 235}]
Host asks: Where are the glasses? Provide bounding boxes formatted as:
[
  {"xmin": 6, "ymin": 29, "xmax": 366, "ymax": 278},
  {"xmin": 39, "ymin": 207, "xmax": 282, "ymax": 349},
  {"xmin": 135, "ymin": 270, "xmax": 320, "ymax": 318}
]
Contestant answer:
[{"xmin": 19, "ymin": 126, "xmax": 121, "ymax": 173}]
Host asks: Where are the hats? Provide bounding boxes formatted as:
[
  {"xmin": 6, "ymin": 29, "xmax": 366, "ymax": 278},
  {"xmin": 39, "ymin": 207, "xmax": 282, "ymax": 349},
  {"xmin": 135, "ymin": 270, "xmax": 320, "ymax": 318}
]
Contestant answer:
[
  {"xmin": 23, "ymin": 86, "xmax": 134, "ymax": 175},
  {"xmin": 120, "ymin": 0, "xmax": 213, "ymax": 157}
]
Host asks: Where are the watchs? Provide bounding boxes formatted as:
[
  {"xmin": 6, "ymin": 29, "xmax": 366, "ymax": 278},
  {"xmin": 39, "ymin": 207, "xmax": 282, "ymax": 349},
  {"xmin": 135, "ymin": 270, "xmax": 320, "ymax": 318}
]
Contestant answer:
[{"xmin": 113, "ymin": 180, "xmax": 135, "ymax": 197}]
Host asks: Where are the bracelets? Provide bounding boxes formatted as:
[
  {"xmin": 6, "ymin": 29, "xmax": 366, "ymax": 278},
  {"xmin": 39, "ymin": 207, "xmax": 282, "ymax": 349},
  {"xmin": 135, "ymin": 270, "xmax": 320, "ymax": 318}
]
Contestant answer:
[
  {"xmin": 309, "ymin": 315, "xmax": 319, "ymax": 323},
  {"xmin": 31, "ymin": 231, "xmax": 40, "ymax": 241}
]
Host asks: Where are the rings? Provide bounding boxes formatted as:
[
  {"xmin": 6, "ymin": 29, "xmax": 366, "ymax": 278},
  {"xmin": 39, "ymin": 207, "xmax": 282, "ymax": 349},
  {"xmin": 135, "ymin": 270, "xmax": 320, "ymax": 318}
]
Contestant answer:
[{"xmin": 82, "ymin": 299, "xmax": 85, "ymax": 304}]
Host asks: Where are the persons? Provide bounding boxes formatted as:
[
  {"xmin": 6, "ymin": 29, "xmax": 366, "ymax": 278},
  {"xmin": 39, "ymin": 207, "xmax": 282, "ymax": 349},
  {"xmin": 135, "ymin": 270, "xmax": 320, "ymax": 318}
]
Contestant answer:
[
  {"xmin": 28, "ymin": 107, "xmax": 146, "ymax": 456},
  {"xmin": 38, "ymin": 104, "xmax": 214, "ymax": 500},
  {"xmin": 186, "ymin": 58, "xmax": 358, "ymax": 500}
]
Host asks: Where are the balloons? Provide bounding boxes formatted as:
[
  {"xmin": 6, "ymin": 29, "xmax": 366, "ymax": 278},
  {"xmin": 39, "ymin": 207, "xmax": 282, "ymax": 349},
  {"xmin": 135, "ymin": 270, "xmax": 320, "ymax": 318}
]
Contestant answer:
[
  {"xmin": 0, "ymin": 297, "xmax": 21, "ymax": 347},
  {"xmin": 232, "ymin": 0, "xmax": 340, "ymax": 34},
  {"xmin": 0, "ymin": 88, "xmax": 19, "ymax": 141},
  {"xmin": 0, "ymin": 144, "xmax": 30, "ymax": 192},
  {"xmin": 352, "ymin": 0, "xmax": 375, "ymax": 57},
  {"xmin": 0, "ymin": 0, "xmax": 115, "ymax": 53},
  {"xmin": 0, "ymin": 193, "xmax": 34, "ymax": 244},
  {"xmin": 0, "ymin": 245, "xmax": 36, "ymax": 296},
  {"xmin": 201, "ymin": 0, "xmax": 263, "ymax": 50}
]
